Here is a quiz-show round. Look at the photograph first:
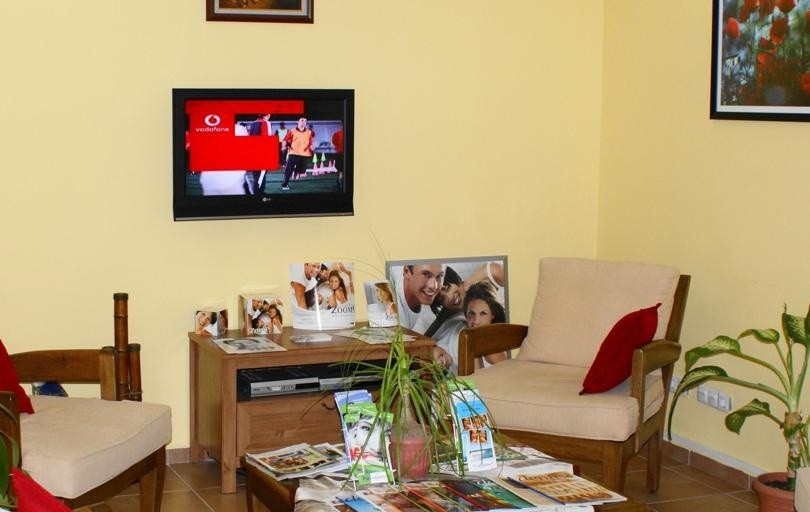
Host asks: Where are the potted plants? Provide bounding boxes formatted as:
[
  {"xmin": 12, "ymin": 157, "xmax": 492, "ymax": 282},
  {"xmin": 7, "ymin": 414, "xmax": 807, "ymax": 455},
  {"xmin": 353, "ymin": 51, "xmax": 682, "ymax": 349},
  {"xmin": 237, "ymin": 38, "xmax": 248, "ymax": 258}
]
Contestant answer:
[
  {"xmin": 299, "ymin": 230, "xmax": 528, "ymax": 493},
  {"xmin": 668, "ymin": 302, "xmax": 810, "ymax": 512}
]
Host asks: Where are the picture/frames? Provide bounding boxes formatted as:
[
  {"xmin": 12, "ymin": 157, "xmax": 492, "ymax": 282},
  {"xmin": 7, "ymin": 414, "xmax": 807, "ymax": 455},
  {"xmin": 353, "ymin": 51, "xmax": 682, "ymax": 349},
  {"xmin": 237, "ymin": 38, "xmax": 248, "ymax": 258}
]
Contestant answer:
[
  {"xmin": 206, "ymin": 0, "xmax": 313, "ymax": 23},
  {"xmin": 710, "ymin": 0, "xmax": 810, "ymax": 122}
]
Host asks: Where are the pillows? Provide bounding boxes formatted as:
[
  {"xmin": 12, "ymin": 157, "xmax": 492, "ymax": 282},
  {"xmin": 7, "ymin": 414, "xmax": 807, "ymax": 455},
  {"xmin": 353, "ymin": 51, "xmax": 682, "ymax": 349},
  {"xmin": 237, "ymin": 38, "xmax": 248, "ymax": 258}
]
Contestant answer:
[
  {"xmin": 0, "ymin": 339, "xmax": 35, "ymax": 414},
  {"xmin": 579, "ymin": 303, "xmax": 663, "ymax": 396},
  {"xmin": 513, "ymin": 257, "xmax": 680, "ymax": 376}
]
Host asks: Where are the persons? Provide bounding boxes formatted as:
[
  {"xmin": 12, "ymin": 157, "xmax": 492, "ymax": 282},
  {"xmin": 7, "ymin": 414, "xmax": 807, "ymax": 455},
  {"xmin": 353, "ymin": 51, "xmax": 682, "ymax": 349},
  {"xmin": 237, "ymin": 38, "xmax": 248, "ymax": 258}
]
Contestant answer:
[
  {"xmin": 190, "ymin": 259, "xmax": 505, "ymax": 375},
  {"xmin": 201, "ymin": 106, "xmax": 343, "ymax": 197}
]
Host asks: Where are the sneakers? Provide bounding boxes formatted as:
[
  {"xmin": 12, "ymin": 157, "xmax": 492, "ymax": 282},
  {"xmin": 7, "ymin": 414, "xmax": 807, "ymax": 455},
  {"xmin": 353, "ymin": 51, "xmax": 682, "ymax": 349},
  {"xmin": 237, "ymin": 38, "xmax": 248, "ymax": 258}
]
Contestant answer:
[{"xmin": 282, "ymin": 184, "xmax": 290, "ymax": 191}]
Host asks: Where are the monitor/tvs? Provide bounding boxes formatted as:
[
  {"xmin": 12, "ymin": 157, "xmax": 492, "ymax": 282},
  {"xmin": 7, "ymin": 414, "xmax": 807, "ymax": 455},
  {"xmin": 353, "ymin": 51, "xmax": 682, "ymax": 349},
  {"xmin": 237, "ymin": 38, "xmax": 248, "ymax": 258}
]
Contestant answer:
[{"xmin": 172, "ymin": 88, "xmax": 353, "ymax": 221}]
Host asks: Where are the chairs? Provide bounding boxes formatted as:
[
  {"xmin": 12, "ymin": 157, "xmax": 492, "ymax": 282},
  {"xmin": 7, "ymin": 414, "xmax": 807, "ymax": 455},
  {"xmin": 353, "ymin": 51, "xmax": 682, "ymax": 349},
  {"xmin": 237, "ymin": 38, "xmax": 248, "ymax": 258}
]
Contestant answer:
[
  {"xmin": 436, "ymin": 275, "xmax": 691, "ymax": 493},
  {"xmin": 0, "ymin": 293, "xmax": 172, "ymax": 512}
]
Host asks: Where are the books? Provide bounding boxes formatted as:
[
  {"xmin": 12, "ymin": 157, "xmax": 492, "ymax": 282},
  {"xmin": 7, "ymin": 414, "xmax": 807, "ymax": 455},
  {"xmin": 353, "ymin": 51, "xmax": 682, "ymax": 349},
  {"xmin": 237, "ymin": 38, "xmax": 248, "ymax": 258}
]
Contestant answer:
[{"xmin": 242, "ymin": 378, "xmax": 627, "ymax": 512}]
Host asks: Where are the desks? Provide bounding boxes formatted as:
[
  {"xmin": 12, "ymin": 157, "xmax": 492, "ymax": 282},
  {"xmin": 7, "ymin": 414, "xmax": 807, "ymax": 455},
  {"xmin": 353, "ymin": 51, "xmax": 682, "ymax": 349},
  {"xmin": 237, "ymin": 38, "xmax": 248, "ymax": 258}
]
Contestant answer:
[{"xmin": 188, "ymin": 321, "xmax": 437, "ymax": 494}]
